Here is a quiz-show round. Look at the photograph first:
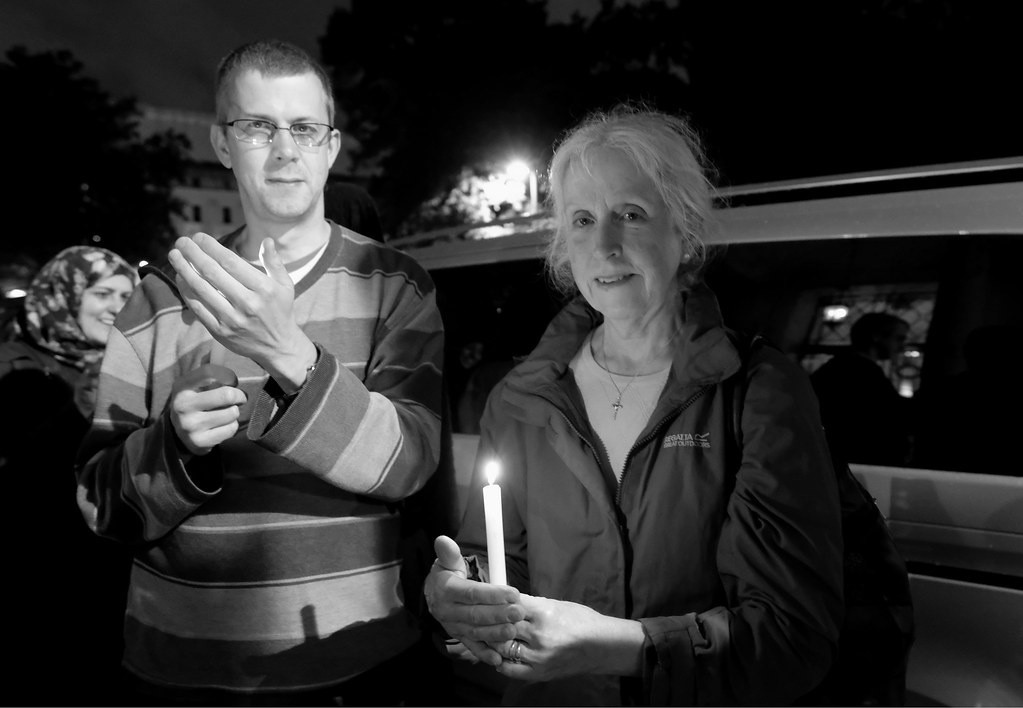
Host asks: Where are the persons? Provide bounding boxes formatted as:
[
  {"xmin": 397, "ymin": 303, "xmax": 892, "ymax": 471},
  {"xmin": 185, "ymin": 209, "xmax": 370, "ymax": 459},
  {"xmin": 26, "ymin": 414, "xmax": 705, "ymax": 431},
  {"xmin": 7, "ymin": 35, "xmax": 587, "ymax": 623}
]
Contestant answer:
[
  {"xmin": 1, "ymin": 245, "xmax": 142, "ymax": 708},
  {"xmin": 73, "ymin": 39, "xmax": 447, "ymax": 708},
  {"xmin": 808, "ymin": 309, "xmax": 1023, "ymax": 479},
  {"xmin": 423, "ymin": 99, "xmax": 844, "ymax": 707}
]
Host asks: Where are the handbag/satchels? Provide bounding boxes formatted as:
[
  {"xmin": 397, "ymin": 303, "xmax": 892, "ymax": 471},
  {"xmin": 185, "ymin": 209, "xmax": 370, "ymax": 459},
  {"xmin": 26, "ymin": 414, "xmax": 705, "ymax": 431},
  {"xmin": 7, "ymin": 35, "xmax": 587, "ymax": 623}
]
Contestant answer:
[{"xmin": 726, "ymin": 336, "xmax": 915, "ymax": 708}]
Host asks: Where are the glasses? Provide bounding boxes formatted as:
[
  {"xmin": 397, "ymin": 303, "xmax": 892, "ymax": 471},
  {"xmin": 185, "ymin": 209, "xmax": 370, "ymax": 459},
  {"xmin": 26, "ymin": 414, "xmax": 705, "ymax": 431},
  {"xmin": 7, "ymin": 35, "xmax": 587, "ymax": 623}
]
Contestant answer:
[{"xmin": 221, "ymin": 118, "xmax": 334, "ymax": 147}]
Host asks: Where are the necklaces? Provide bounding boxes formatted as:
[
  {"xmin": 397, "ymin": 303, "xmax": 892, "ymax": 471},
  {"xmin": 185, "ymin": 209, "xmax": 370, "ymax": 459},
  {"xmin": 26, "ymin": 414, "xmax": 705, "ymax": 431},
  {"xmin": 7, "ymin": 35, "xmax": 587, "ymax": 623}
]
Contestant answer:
[{"xmin": 600, "ymin": 319, "xmax": 684, "ymax": 420}]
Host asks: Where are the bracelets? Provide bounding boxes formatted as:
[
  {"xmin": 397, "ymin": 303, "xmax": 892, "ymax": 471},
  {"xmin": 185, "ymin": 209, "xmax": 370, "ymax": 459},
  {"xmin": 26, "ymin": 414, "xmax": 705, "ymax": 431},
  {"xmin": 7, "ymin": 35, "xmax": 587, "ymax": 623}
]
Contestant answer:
[{"xmin": 282, "ymin": 341, "xmax": 321, "ymax": 404}]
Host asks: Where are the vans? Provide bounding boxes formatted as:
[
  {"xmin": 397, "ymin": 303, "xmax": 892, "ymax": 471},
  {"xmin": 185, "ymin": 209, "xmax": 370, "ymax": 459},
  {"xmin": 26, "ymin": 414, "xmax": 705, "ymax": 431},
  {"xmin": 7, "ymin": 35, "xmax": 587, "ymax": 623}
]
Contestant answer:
[{"xmin": 397, "ymin": 148, "xmax": 1021, "ymax": 707}]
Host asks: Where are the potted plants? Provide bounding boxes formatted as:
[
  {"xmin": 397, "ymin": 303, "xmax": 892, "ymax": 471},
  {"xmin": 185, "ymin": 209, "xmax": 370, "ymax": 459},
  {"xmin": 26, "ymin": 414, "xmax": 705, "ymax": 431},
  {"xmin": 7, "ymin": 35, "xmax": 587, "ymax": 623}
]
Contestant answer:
[{"xmin": 210, "ymin": 334, "xmax": 225, "ymax": 365}]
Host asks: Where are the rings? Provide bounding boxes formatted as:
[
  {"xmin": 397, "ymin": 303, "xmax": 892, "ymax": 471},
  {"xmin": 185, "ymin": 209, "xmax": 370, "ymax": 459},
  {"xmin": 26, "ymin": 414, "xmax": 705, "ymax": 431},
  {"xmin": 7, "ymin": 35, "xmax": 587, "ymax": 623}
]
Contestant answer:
[
  {"xmin": 515, "ymin": 642, "xmax": 522, "ymax": 664},
  {"xmin": 509, "ymin": 640, "xmax": 517, "ymax": 662}
]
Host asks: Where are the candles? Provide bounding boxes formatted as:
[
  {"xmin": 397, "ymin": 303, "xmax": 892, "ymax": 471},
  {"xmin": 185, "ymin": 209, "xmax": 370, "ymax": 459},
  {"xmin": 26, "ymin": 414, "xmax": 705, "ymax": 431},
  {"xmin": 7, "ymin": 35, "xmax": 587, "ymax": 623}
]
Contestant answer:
[{"xmin": 482, "ymin": 461, "xmax": 507, "ymax": 584}]
